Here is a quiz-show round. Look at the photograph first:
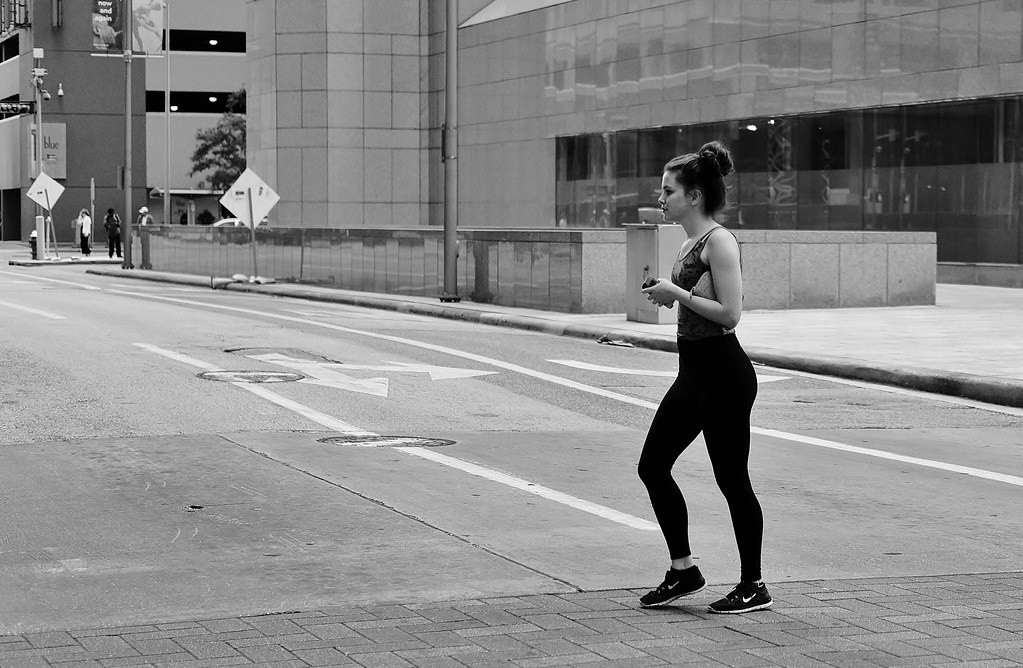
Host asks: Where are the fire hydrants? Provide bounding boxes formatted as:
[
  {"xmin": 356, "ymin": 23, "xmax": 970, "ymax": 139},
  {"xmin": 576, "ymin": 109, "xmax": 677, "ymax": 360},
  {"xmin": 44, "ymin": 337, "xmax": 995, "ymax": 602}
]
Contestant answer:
[{"xmin": 29, "ymin": 230, "xmax": 37, "ymax": 260}]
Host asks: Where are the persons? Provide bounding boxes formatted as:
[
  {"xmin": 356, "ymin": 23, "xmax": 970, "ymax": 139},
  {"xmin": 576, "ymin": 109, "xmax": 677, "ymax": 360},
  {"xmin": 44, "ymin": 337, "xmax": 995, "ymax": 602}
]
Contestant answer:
[
  {"xmin": 638, "ymin": 140, "xmax": 773, "ymax": 613},
  {"xmin": 565, "ymin": 202, "xmax": 607, "ymax": 228},
  {"xmin": 78, "ymin": 208, "xmax": 91, "ymax": 256},
  {"xmin": 103, "ymin": 208, "xmax": 122, "ymax": 258},
  {"xmin": 136, "ymin": 207, "xmax": 155, "ymax": 231},
  {"xmin": 178, "ymin": 209, "xmax": 187, "ymax": 225}
]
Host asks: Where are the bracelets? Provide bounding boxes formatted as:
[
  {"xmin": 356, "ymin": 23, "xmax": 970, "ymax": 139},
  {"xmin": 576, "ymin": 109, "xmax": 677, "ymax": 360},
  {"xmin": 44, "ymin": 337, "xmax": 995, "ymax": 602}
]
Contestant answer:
[{"xmin": 684, "ymin": 290, "xmax": 694, "ymax": 307}]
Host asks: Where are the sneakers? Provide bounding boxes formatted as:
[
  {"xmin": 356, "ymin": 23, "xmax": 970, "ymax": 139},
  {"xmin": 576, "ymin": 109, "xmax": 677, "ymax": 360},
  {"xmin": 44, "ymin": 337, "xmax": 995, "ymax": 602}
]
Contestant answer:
[
  {"xmin": 638, "ymin": 565, "xmax": 706, "ymax": 607},
  {"xmin": 707, "ymin": 581, "xmax": 773, "ymax": 614}
]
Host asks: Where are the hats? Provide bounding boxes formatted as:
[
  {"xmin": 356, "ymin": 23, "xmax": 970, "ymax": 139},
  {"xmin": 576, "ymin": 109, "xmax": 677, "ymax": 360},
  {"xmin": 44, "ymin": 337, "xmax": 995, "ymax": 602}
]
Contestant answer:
[{"xmin": 138, "ymin": 207, "xmax": 148, "ymax": 213}]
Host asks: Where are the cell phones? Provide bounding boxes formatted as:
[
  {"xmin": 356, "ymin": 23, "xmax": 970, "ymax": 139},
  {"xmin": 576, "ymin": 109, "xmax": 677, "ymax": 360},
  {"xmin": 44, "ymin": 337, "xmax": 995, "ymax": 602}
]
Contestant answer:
[{"xmin": 641, "ymin": 276, "xmax": 676, "ymax": 309}]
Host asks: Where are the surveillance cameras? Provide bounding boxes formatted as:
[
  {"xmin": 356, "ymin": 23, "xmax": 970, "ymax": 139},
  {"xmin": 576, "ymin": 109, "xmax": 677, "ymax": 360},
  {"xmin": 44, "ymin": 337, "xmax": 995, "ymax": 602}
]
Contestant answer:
[
  {"xmin": 43, "ymin": 93, "xmax": 51, "ymax": 101},
  {"xmin": 57, "ymin": 89, "xmax": 64, "ymax": 97}
]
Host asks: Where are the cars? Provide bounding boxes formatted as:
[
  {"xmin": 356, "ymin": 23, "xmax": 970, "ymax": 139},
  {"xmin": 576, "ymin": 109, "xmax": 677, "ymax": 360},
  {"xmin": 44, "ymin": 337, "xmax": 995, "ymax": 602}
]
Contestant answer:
[{"xmin": 212, "ymin": 217, "xmax": 268, "ymax": 228}]
